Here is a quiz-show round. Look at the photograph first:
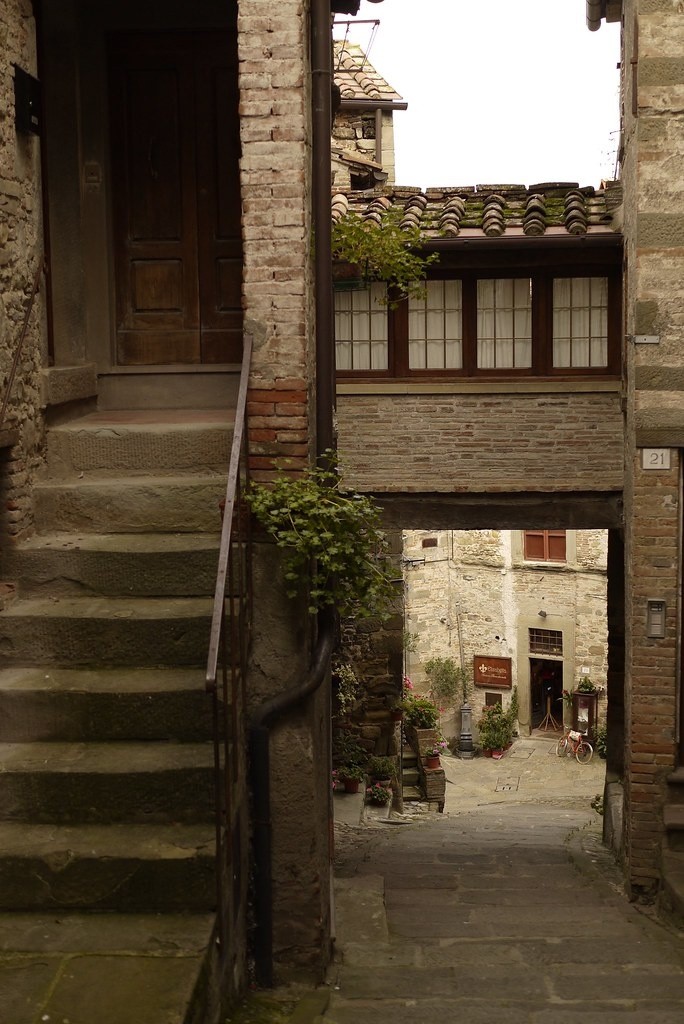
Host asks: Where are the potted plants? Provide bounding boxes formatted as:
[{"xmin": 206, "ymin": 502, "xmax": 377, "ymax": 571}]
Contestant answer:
[
  {"xmin": 592, "ymin": 723, "xmax": 607, "ymax": 760},
  {"xmin": 368, "ymin": 756, "xmax": 398, "ymax": 788},
  {"xmin": 578, "ymin": 676, "xmax": 596, "ymax": 693},
  {"xmin": 332, "ymin": 205, "xmax": 441, "ymax": 311},
  {"xmin": 330, "ymin": 663, "xmax": 360, "ymax": 720},
  {"xmin": 338, "ymin": 764, "xmax": 363, "ymax": 793},
  {"xmin": 218, "ymin": 447, "xmax": 405, "ymax": 622}
]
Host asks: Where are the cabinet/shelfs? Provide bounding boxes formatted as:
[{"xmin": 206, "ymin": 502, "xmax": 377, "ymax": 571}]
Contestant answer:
[{"xmin": 572, "ymin": 689, "xmax": 600, "ymax": 751}]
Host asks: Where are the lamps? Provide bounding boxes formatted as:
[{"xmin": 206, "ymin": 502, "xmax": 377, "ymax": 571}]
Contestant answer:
[{"xmin": 537, "ymin": 610, "xmax": 547, "ymax": 618}]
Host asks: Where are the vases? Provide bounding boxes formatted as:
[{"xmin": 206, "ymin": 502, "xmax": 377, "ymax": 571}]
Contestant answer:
[
  {"xmin": 413, "ymin": 713, "xmax": 429, "ymax": 725},
  {"xmin": 492, "ymin": 749, "xmax": 504, "ymax": 760},
  {"xmin": 483, "ymin": 748, "xmax": 493, "ymax": 758},
  {"xmin": 372, "ymin": 799, "xmax": 388, "ymax": 807},
  {"xmin": 503, "ymin": 741, "xmax": 514, "ymax": 751},
  {"xmin": 426, "ymin": 757, "xmax": 439, "ymax": 769}
]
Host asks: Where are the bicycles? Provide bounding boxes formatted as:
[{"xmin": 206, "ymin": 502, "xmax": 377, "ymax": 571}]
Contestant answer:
[{"xmin": 555, "ymin": 725, "xmax": 594, "ymax": 765}]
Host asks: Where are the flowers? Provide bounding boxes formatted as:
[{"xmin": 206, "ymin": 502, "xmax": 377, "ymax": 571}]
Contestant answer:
[
  {"xmin": 426, "ymin": 737, "xmax": 450, "ymax": 757},
  {"xmin": 332, "ymin": 769, "xmax": 340, "ymax": 791},
  {"xmin": 362, "ymin": 783, "xmax": 394, "ymax": 803},
  {"xmin": 475, "ymin": 701, "xmax": 512, "ymax": 750},
  {"xmin": 400, "ymin": 675, "xmax": 444, "ymax": 729}
]
